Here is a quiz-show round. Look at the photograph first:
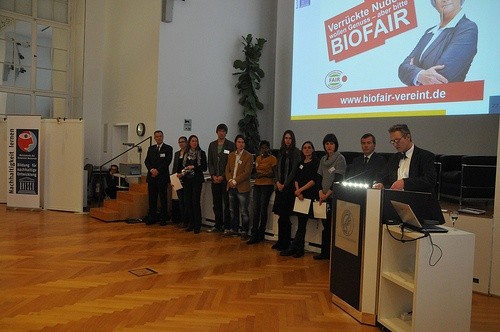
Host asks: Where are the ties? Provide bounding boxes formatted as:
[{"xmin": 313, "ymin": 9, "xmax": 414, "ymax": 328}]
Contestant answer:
[
  {"xmin": 364, "ymin": 157, "xmax": 368, "ymax": 163},
  {"xmin": 157, "ymin": 146, "xmax": 159, "ymax": 150}
]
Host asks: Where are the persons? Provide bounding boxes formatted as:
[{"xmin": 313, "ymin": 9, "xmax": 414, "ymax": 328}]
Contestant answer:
[
  {"xmin": 397, "ymin": 0, "xmax": 478, "ymax": 85},
  {"xmin": 208, "ymin": 124, "xmax": 237, "ymax": 237},
  {"xmin": 171, "ymin": 137, "xmax": 189, "ymax": 228},
  {"xmin": 278, "ymin": 141, "xmax": 322, "ymax": 257},
  {"xmin": 313, "ymin": 134, "xmax": 346, "ymax": 259},
  {"xmin": 176, "ymin": 135, "xmax": 207, "ymax": 233},
  {"xmin": 224, "ymin": 133, "xmax": 254, "ymax": 239},
  {"xmin": 105, "ymin": 165, "xmax": 127, "ymax": 199},
  {"xmin": 370, "ymin": 123, "xmax": 437, "ymax": 191},
  {"xmin": 272, "ymin": 130, "xmax": 304, "ymax": 250},
  {"xmin": 144, "ymin": 131, "xmax": 173, "ymax": 226},
  {"xmin": 245, "ymin": 140, "xmax": 278, "ymax": 245},
  {"xmin": 347, "ymin": 133, "xmax": 391, "ymax": 186}
]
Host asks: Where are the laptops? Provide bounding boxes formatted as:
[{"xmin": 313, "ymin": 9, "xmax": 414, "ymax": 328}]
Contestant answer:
[{"xmin": 390, "ymin": 201, "xmax": 449, "ymax": 234}]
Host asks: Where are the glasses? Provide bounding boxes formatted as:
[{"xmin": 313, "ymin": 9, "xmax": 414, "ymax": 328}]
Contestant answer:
[{"xmin": 389, "ymin": 135, "xmax": 405, "ymax": 144}]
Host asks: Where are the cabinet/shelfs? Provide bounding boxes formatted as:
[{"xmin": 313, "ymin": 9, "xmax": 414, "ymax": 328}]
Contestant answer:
[{"xmin": 378, "ymin": 224, "xmax": 476, "ymax": 332}]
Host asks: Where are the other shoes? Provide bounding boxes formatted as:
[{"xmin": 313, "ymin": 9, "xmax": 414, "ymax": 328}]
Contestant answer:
[
  {"xmin": 206, "ymin": 226, "xmax": 220, "ymax": 232},
  {"xmin": 194, "ymin": 227, "xmax": 200, "ymax": 233},
  {"xmin": 146, "ymin": 219, "xmax": 157, "ymax": 225},
  {"xmin": 230, "ymin": 229, "xmax": 239, "ymax": 236},
  {"xmin": 222, "ymin": 228, "xmax": 230, "ymax": 235},
  {"xmin": 241, "ymin": 231, "xmax": 248, "ymax": 241},
  {"xmin": 313, "ymin": 254, "xmax": 330, "ymax": 259},
  {"xmin": 272, "ymin": 242, "xmax": 291, "ymax": 250},
  {"xmin": 248, "ymin": 228, "xmax": 264, "ymax": 244},
  {"xmin": 280, "ymin": 249, "xmax": 305, "ymax": 257},
  {"xmin": 185, "ymin": 226, "xmax": 193, "ymax": 231},
  {"xmin": 160, "ymin": 219, "xmax": 167, "ymax": 226}
]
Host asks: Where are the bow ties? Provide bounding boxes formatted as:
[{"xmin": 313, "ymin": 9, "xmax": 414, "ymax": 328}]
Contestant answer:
[{"xmin": 400, "ymin": 154, "xmax": 406, "ymax": 160}]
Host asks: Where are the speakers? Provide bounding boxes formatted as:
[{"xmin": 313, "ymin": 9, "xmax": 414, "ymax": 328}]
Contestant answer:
[{"xmin": 162, "ymin": 0, "xmax": 174, "ymax": 23}]
